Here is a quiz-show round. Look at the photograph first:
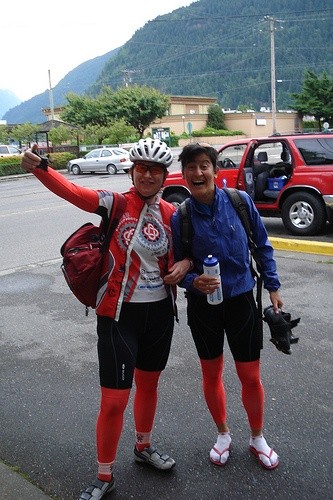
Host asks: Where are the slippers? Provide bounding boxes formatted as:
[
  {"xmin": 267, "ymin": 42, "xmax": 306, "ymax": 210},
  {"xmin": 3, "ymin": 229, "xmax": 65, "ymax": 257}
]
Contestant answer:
[
  {"xmin": 210, "ymin": 442, "xmax": 232, "ymax": 466},
  {"xmin": 250, "ymin": 444, "xmax": 279, "ymax": 469}
]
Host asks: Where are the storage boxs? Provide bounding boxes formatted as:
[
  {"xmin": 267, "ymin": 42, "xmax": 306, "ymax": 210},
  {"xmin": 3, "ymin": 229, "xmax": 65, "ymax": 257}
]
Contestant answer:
[{"xmin": 268, "ymin": 176, "xmax": 288, "ymax": 190}]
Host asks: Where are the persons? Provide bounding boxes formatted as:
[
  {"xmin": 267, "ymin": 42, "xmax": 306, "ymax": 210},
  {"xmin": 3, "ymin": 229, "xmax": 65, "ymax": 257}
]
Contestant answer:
[
  {"xmin": 171, "ymin": 144, "xmax": 283, "ymax": 467},
  {"xmin": 21, "ymin": 137, "xmax": 194, "ymax": 500}
]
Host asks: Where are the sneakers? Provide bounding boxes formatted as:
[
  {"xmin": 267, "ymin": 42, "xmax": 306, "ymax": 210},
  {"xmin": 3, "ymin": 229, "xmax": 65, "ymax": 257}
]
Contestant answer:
[
  {"xmin": 134, "ymin": 445, "xmax": 176, "ymax": 472},
  {"xmin": 263, "ymin": 305, "xmax": 301, "ymax": 355},
  {"xmin": 77, "ymin": 472, "xmax": 115, "ymax": 500}
]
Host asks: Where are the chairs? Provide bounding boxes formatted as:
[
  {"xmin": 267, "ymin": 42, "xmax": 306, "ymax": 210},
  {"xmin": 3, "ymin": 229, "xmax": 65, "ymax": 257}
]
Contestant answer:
[
  {"xmin": 274, "ymin": 152, "xmax": 291, "ymax": 174},
  {"xmin": 254, "ymin": 152, "xmax": 269, "ymax": 178}
]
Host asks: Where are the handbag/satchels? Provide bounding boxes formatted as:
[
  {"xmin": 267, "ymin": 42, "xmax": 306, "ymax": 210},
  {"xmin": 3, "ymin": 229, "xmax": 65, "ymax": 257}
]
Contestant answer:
[{"xmin": 60, "ymin": 191, "xmax": 126, "ymax": 316}]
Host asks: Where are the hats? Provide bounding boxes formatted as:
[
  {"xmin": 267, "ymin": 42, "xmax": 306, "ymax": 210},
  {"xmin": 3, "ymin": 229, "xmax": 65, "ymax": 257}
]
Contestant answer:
[{"xmin": 180, "ymin": 143, "xmax": 219, "ymax": 162}]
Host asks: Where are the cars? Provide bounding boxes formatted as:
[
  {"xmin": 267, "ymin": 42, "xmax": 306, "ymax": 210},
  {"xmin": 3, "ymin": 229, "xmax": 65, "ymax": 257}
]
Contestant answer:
[
  {"xmin": 161, "ymin": 135, "xmax": 332, "ymax": 236},
  {"xmin": 67, "ymin": 148, "xmax": 134, "ymax": 175},
  {"xmin": 0, "ymin": 143, "xmax": 49, "ymax": 158}
]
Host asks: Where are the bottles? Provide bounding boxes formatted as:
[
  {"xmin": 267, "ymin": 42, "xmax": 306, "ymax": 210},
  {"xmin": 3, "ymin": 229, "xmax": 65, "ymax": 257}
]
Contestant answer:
[{"xmin": 203, "ymin": 255, "xmax": 223, "ymax": 305}]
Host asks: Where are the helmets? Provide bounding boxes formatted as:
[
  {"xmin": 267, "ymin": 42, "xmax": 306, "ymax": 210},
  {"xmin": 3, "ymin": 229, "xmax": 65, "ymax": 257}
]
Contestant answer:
[{"xmin": 129, "ymin": 137, "xmax": 174, "ymax": 169}]
